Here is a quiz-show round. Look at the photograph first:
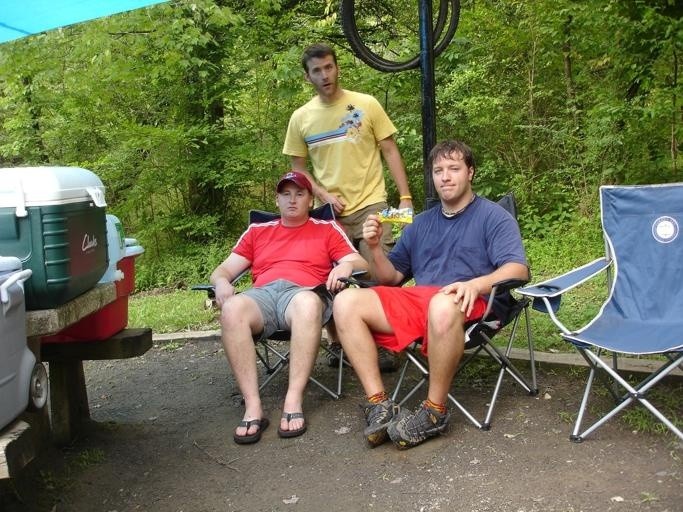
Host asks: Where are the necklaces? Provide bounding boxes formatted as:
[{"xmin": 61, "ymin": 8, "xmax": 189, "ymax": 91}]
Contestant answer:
[{"xmin": 441, "ymin": 195, "xmax": 474, "ymax": 214}]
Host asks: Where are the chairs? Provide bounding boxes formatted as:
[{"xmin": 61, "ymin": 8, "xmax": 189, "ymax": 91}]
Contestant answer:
[
  {"xmin": 191, "ymin": 200, "xmax": 369, "ymax": 405},
  {"xmin": 513, "ymin": 181, "xmax": 683, "ymax": 443},
  {"xmin": 337, "ymin": 190, "xmax": 540, "ymax": 431}
]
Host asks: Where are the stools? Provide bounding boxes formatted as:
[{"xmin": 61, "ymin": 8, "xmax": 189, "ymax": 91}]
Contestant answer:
[{"xmin": 40, "ymin": 327, "xmax": 152, "ymax": 448}]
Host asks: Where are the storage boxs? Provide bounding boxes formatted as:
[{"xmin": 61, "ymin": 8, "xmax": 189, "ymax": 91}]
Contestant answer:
[
  {"xmin": 0, "ymin": 255, "xmax": 49, "ymax": 432},
  {"xmin": 41, "ymin": 238, "xmax": 146, "ymax": 344},
  {"xmin": 97, "ymin": 214, "xmax": 127, "ymax": 283},
  {"xmin": 0, "ymin": 165, "xmax": 111, "ymax": 312}
]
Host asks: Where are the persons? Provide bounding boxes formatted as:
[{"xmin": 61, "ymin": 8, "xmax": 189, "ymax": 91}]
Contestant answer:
[
  {"xmin": 209, "ymin": 171, "xmax": 371, "ymax": 444},
  {"xmin": 282, "ymin": 42, "xmax": 416, "ymax": 373},
  {"xmin": 332, "ymin": 140, "xmax": 530, "ymax": 451}
]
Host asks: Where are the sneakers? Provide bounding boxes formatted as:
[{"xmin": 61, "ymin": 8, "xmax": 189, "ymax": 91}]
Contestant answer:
[
  {"xmin": 359, "ymin": 399, "xmax": 411, "ymax": 448},
  {"xmin": 326, "ymin": 343, "xmax": 349, "ymax": 367},
  {"xmin": 386, "ymin": 400, "xmax": 451, "ymax": 451},
  {"xmin": 375, "ymin": 346, "xmax": 402, "ymax": 373}
]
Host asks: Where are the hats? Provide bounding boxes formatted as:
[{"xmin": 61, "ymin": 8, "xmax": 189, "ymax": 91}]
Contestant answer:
[{"xmin": 275, "ymin": 171, "xmax": 313, "ymax": 195}]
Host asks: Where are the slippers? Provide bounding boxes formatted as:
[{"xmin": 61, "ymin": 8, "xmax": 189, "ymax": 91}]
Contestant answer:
[
  {"xmin": 233, "ymin": 418, "xmax": 269, "ymax": 445},
  {"xmin": 277, "ymin": 405, "xmax": 307, "ymax": 439}
]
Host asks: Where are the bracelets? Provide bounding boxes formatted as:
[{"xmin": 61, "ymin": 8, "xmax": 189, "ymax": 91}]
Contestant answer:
[{"xmin": 399, "ymin": 196, "xmax": 412, "ymax": 200}]
